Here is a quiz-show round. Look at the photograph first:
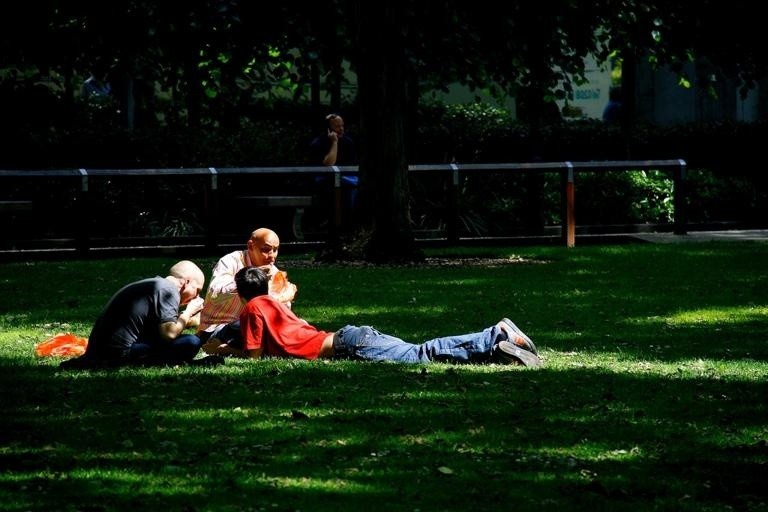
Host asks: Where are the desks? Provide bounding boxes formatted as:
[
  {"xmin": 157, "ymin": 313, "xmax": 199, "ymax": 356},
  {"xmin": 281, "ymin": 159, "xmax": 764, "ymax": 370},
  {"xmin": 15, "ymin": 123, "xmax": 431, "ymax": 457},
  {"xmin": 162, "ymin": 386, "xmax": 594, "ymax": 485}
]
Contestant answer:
[
  {"xmin": 0, "ymin": 200, "xmax": 33, "ymax": 214},
  {"xmin": 238, "ymin": 194, "xmax": 312, "ymax": 241}
]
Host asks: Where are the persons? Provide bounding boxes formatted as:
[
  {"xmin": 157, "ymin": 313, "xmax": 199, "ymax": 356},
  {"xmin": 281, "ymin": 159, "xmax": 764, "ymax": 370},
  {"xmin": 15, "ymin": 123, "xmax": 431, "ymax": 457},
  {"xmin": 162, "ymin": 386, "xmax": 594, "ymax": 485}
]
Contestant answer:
[
  {"xmin": 216, "ymin": 265, "xmax": 544, "ymax": 369},
  {"xmin": 301, "ymin": 113, "xmax": 360, "ymax": 254},
  {"xmin": 195, "ymin": 228, "xmax": 297, "ymax": 353},
  {"xmin": 79, "ymin": 258, "xmax": 225, "ymax": 369},
  {"xmin": 603, "ymin": 86, "xmax": 622, "ymax": 125}
]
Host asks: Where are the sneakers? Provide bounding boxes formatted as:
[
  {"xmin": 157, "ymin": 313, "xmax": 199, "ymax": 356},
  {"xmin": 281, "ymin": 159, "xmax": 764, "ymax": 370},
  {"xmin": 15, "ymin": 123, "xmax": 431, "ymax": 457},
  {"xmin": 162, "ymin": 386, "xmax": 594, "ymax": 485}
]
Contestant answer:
[
  {"xmin": 498, "ymin": 341, "xmax": 540, "ymax": 367},
  {"xmin": 497, "ymin": 318, "xmax": 537, "ymax": 355}
]
